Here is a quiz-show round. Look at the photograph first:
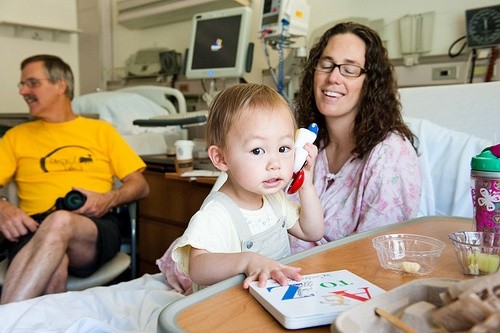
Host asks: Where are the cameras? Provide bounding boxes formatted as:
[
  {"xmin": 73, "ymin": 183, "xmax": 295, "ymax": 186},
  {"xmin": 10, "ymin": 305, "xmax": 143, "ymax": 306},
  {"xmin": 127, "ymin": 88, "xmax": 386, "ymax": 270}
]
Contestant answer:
[{"xmin": 56, "ymin": 190, "xmax": 86, "ymax": 211}]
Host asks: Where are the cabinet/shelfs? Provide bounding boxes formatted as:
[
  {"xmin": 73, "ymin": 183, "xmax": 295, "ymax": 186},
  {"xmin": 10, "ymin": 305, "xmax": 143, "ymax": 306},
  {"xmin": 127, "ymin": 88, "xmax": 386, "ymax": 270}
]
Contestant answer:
[{"xmin": 135, "ymin": 153, "xmax": 220, "ymax": 279}]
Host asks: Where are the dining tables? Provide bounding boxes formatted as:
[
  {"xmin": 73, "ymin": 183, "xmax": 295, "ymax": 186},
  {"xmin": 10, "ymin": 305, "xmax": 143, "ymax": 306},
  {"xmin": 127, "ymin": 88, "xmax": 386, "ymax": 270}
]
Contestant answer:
[{"xmin": 160, "ymin": 217, "xmax": 500, "ymax": 333}]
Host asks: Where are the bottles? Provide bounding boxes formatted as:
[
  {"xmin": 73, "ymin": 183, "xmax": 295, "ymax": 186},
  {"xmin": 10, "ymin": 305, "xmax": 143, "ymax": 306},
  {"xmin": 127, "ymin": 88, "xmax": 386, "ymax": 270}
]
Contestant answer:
[{"xmin": 470, "ymin": 146, "xmax": 500, "ymax": 253}]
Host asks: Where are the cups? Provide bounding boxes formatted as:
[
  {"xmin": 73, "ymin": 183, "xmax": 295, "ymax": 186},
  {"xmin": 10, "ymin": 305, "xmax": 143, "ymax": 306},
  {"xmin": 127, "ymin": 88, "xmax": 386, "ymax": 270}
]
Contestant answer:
[{"xmin": 174, "ymin": 139, "xmax": 194, "ymax": 172}]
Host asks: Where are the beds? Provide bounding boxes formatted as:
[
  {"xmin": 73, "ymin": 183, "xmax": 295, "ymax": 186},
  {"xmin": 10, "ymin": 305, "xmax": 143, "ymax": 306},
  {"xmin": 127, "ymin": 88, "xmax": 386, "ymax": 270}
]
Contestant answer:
[{"xmin": 70, "ymin": 84, "xmax": 189, "ymax": 190}]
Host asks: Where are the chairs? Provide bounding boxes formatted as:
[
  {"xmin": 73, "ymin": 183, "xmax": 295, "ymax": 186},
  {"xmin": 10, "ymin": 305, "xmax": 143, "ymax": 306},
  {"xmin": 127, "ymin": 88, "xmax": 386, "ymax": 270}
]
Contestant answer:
[{"xmin": 0, "ymin": 113, "xmax": 130, "ymax": 291}]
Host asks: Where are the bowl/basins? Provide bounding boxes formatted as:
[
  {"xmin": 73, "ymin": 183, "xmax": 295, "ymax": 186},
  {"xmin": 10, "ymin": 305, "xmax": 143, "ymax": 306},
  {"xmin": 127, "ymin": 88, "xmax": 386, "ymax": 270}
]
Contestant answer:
[
  {"xmin": 372, "ymin": 234, "xmax": 447, "ymax": 276},
  {"xmin": 447, "ymin": 232, "xmax": 500, "ymax": 276}
]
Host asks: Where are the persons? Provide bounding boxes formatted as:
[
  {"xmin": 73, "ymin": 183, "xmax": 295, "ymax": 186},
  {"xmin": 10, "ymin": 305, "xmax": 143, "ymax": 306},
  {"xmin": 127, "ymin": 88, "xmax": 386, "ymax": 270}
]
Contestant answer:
[
  {"xmin": 0, "ymin": 54, "xmax": 150, "ymax": 305},
  {"xmin": 170, "ymin": 82, "xmax": 325, "ymax": 294},
  {"xmin": 156, "ymin": 21, "xmax": 425, "ymax": 294}
]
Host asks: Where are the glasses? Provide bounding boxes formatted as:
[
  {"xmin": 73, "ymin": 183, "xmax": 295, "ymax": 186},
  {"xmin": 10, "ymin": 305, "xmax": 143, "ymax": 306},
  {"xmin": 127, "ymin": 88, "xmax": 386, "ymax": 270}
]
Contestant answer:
[
  {"xmin": 313, "ymin": 59, "xmax": 369, "ymax": 77},
  {"xmin": 17, "ymin": 79, "xmax": 64, "ymax": 90}
]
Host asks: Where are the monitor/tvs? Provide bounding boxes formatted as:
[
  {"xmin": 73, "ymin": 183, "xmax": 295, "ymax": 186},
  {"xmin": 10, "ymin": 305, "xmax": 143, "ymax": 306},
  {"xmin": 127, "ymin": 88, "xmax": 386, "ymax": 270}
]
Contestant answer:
[{"xmin": 185, "ymin": 6, "xmax": 252, "ymax": 91}]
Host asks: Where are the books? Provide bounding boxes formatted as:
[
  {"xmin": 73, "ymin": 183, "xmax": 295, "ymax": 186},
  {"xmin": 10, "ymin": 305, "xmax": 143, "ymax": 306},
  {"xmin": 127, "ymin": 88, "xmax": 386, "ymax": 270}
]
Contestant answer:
[{"xmin": 250, "ymin": 269, "xmax": 388, "ymax": 329}]
